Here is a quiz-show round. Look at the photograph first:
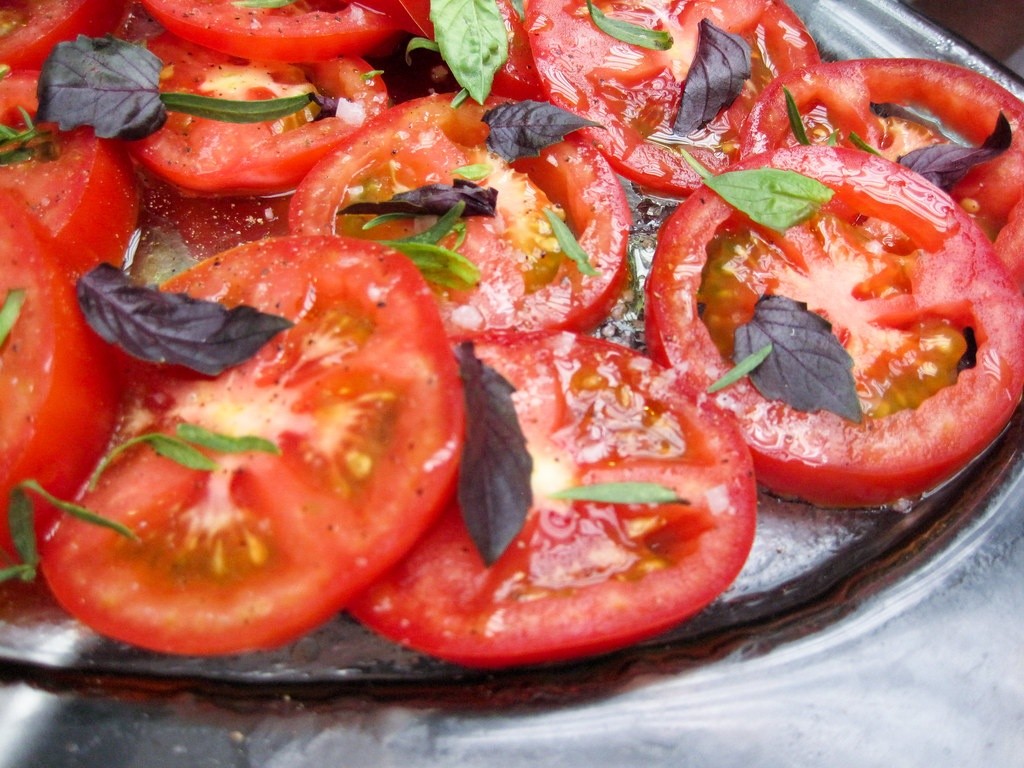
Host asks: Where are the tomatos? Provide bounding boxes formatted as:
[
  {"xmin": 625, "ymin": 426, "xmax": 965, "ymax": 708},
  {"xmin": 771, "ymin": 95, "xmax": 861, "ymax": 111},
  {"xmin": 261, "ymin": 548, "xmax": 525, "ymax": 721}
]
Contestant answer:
[{"xmin": 0, "ymin": 0, "xmax": 1024, "ymax": 663}]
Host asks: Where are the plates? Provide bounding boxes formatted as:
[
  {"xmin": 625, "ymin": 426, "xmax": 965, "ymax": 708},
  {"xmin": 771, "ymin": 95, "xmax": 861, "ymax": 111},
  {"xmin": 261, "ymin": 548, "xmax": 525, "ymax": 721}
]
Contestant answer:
[{"xmin": 0, "ymin": 0, "xmax": 1024, "ymax": 768}]
